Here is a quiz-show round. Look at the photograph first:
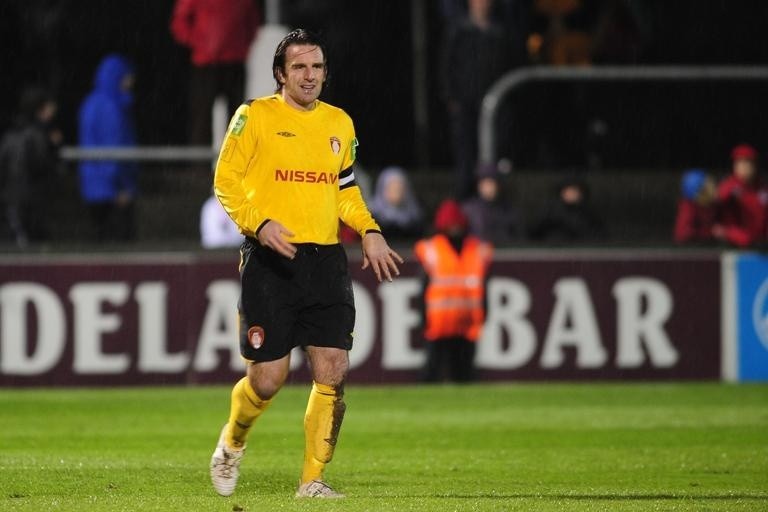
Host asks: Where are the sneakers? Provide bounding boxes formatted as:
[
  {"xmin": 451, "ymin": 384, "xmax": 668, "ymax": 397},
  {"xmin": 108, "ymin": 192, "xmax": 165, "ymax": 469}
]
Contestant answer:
[
  {"xmin": 210, "ymin": 423, "xmax": 246, "ymax": 496},
  {"xmin": 295, "ymin": 480, "xmax": 346, "ymax": 497}
]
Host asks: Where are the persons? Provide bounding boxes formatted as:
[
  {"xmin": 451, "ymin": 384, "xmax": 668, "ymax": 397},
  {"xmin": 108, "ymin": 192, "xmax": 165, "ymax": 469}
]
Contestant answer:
[
  {"xmin": 338, "ymin": 162, "xmax": 601, "ymax": 245},
  {"xmin": 417, "ymin": 200, "xmax": 498, "ymax": 383},
  {"xmin": 434, "ymin": 1, "xmax": 517, "ymax": 170},
  {"xmin": 0, "ymin": 89, "xmax": 65, "ymax": 248},
  {"xmin": 79, "ymin": 53, "xmax": 142, "ymax": 249},
  {"xmin": 170, "ymin": 0, "xmax": 260, "ymax": 146},
  {"xmin": 211, "ymin": 28, "xmax": 407, "ymax": 502},
  {"xmin": 675, "ymin": 146, "xmax": 768, "ymax": 246},
  {"xmin": 200, "ymin": 192, "xmax": 246, "ymax": 249}
]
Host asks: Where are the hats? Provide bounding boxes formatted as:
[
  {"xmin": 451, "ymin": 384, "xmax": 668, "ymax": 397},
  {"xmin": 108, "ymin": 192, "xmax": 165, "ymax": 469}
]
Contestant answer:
[
  {"xmin": 731, "ymin": 145, "xmax": 758, "ymax": 160},
  {"xmin": 435, "ymin": 199, "xmax": 468, "ymax": 229},
  {"xmin": 682, "ymin": 171, "xmax": 705, "ymax": 199}
]
